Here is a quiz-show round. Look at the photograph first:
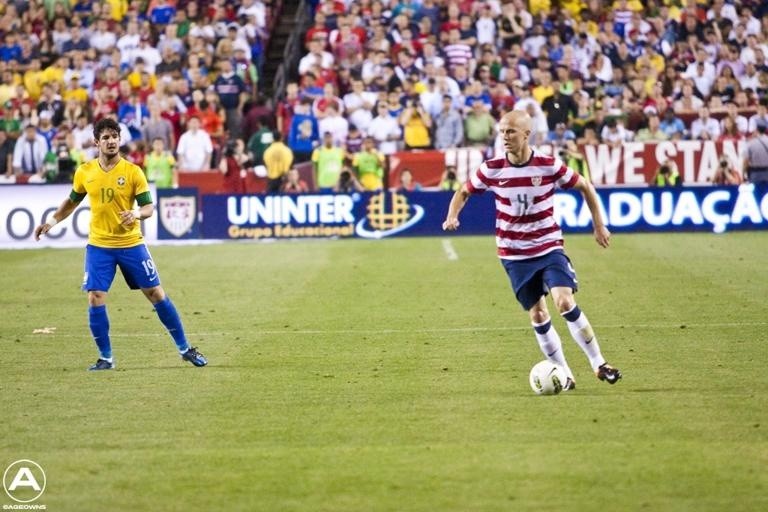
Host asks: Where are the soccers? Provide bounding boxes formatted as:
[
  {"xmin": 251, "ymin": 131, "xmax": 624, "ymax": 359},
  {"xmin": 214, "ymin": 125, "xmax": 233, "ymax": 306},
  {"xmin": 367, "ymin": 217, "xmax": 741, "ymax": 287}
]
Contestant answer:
[{"xmin": 530, "ymin": 360, "xmax": 565, "ymax": 395}]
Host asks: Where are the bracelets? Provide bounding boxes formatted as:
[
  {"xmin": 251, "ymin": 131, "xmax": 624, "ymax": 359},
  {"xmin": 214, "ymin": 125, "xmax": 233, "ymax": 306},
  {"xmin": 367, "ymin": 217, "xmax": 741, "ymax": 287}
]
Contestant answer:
[
  {"xmin": 47, "ymin": 217, "xmax": 58, "ymax": 228},
  {"xmin": 131, "ymin": 210, "xmax": 140, "ymax": 220}
]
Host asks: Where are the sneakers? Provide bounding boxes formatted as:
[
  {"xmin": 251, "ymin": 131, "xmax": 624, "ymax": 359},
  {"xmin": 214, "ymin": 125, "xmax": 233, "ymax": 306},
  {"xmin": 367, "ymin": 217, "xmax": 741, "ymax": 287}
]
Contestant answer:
[
  {"xmin": 179, "ymin": 346, "xmax": 207, "ymax": 366},
  {"xmin": 596, "ymin": 362, "xmax": 622, "ymax": 384},
  {"xmin": 559, "ymin": 374, "xmax": 576, "ymax": 391},
  {"xmin": 87, "ymin": 358, "xmax": 114, "ymax": 370}
]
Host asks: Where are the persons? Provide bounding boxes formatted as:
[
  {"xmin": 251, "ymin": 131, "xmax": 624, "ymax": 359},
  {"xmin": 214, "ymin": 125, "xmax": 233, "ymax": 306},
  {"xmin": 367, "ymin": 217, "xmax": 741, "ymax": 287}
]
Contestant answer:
[
  {"xmin": 34, "ymin": 117, "xmax": 209, "ymax": 371},
  {"xmin": 441, "ymin": 110, "xmax": 623, "ymax": 393},
  {"xmin": 0, "ymin": 0, "xmax": 768, "ymax": 192}
]
